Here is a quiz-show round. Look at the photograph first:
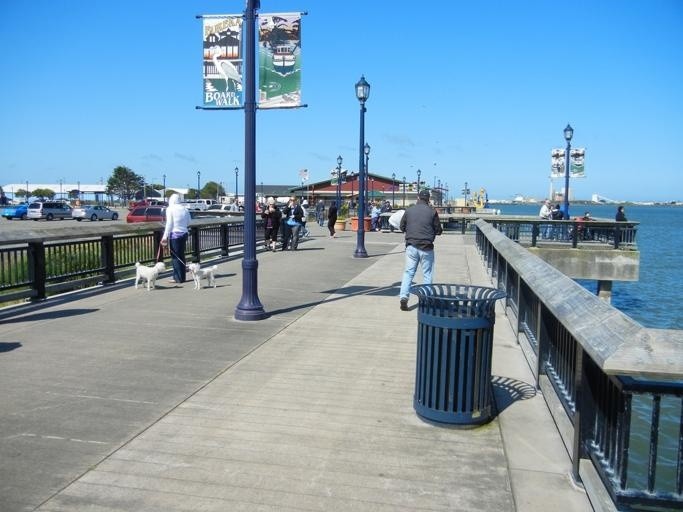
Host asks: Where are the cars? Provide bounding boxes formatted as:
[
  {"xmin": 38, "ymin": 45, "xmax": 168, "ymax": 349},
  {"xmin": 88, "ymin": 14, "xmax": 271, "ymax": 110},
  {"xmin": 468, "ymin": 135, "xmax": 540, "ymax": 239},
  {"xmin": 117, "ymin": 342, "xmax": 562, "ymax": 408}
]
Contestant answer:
[
  {"xmin": 273, "ymin": 202, "xmax": 287, "ymax": 214},
  {"xmin": 180, "ymin": 198, "xmax": 241, "ymax": 212},
  {"xmin": 0, "ymin": 204, "xmax": 29, "ymax": 220},
  {"xmin": 70, "ymin": 204, "xmax": 118, "ymax": 221}
]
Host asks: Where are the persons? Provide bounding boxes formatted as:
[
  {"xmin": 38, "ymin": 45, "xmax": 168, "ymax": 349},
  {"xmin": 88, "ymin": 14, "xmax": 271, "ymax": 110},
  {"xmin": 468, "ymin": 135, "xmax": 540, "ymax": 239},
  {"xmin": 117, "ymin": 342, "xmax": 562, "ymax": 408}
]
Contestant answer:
[
  {"xmin": 548, "ymin": 203, "xmax": 555, "ymax": 221},
  {"xmin": 537, "ymin": 199, "xmax": 551, "ymax": 239},
  {"xmin": 228, "ymin": 196, "xmax": 266, "ymax": 215},
  {"xmin": 326, "ymin": 201, "xmax": 337, "ymax": 239},
  {"xmin": 260, "ymin": 197, "xmax": 282, "ymax": 252},
  {"xmin": 316, "ymin": 199, "xmax": 325, "ymax": 222},
  {"xmin": 157, "ymin": 192, "xmax": 191, "ymax": 283},
  {"xmin": 615, "ymin": 206, "xmax": 628, "ymax": 222},
  {"xmin": 280, "ymin": 195, "xmax": 304, "ymax": 250},
  {"xmin": 550, "ymin": 204, "xmax": 563, "ymax": 239},
  {"xmin": 347, "ymin": 192, "xmax": 393, "ymax": 233},
  {"xmin": 317, "ymin": 207, "xmax": 324, "ymax": 227},
  {"xmin": 396, "ymin": 188, "xmax": 442, "ymax": 311},
  {"xmin": 580, "ymin": 211, "xmax": 593, "ymax": 221}
]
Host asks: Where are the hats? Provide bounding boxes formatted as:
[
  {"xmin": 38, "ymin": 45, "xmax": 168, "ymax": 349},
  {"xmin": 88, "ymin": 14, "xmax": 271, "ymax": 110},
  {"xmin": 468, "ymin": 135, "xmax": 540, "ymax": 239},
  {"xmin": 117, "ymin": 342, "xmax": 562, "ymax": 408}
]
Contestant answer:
[{"xmin": 419, "ymin": 191, "xmax": 430, "ymax": 197}]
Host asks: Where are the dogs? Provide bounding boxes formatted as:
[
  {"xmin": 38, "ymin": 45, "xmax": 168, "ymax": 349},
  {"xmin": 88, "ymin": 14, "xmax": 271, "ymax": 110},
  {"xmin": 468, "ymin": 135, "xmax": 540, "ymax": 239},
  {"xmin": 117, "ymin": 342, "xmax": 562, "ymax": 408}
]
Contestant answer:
[
  {"xmin": 133, "ymin": 261, "xmax": 169, "ymax": 292},
  {"xmin": 187, "ymin": 262, "xmax": 218, "ymax": 290}
]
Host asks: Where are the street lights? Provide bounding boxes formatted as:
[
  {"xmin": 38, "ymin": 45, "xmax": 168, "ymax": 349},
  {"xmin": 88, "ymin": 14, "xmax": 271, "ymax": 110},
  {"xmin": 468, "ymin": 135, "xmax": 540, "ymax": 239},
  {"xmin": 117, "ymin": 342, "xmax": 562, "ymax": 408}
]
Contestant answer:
[
  {"xmin": 234, "ymin": 166, "xmax": 237, "ymax": 206},
  {"xmin": 196, "ymin": 169, "xmax": 200, "ymax": 193},
  {"xmin": 560, "ymin": 123, "xmax": 573, "ymax": 239},
  {"xmin": 371, "ymin": 169, "xmax": 468, "ymax": 206},
  {"xmin": 353, "ymin": 73, "xmax": 369, "ymax": 258},
  {"xmin": 162, "ymin": 173, "xmax": 166, "ymax": 204},
  {"xmin": 361, "ymin": 142, "xmax": 370, "ymax": 217},
  {"xmin": 334, "ymin": 154, "xmax": 342, "ymax": 216},
  {"xmin": 350, "ymin": 171, "xmax": 354, "ymax": 210}
]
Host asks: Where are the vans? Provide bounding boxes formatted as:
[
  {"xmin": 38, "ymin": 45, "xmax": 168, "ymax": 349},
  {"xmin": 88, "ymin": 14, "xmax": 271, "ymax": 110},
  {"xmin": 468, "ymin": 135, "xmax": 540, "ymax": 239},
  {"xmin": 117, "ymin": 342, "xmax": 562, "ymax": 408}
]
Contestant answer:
[{"xmin": 26, "ymin": 201, "xmax": 74, "ymax": 221}]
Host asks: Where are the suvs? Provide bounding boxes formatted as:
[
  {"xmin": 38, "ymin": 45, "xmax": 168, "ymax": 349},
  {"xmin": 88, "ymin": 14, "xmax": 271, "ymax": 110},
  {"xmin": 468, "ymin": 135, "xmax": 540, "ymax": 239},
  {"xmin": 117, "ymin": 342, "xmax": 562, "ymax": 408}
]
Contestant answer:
[{"xmin": 126, "ymin": 205, "xmax": 166, "ymax": 223}]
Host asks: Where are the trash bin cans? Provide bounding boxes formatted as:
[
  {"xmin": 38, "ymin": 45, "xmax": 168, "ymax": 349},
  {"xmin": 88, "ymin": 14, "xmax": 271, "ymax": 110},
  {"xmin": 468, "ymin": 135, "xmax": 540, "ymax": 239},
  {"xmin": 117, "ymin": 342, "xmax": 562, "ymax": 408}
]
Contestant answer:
[
  {"xmin": 410, "ymin": 284, "xmax": 508, "ymax": 425},
  {"xmin": 363, "ymin": 217, "xmax": 372, "ymax": 231},
  {"xmin": 352, "ymin": 217, "xmax": 359, "ymax": 232}
]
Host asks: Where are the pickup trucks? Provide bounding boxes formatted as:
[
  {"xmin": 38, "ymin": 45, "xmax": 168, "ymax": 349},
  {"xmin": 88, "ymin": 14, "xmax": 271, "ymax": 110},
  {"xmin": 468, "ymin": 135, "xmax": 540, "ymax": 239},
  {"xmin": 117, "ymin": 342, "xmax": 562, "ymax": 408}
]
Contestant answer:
[{"xmin": 127, "ymin": 199, "xmax": 167, "ymax": 210}]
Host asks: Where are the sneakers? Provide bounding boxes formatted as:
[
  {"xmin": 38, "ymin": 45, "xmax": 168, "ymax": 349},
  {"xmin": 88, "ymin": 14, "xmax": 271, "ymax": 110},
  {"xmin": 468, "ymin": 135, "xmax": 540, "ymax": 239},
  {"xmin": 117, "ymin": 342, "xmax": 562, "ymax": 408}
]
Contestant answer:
[
  {"xmin": 169, "ymin": 279, "xmax": 181, "ymax": 283},
  {"xmin": 330, "ymin": 236, "xmax": 334, "ymax": 238},
  {"xmin": 332, "ymin": 232, "xmax": 336, "ymax": 237},
  {"xmin": 400, "ymin": 297, "xmax": 409, "ymax": 310}
]
